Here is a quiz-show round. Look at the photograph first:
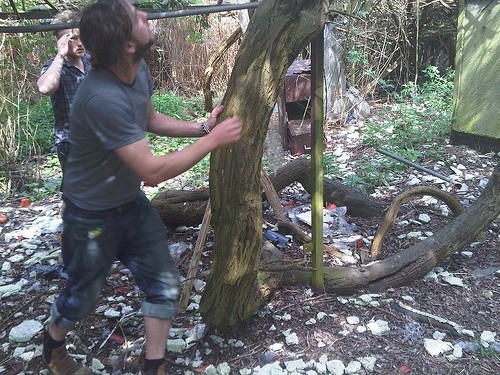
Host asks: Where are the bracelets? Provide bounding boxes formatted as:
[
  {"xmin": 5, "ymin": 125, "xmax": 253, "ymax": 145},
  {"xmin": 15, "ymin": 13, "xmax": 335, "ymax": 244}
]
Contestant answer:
[
  {"xmin": 200, "ymin": 121, "xmax": 209, "ymax": 134},
  {"xmin": 57, "ymin": 53, "xmax": 66, "ymax": 58}
]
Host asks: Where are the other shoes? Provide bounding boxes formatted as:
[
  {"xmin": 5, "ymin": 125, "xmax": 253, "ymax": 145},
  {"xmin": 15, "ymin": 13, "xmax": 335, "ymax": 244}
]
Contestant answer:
[
  {"xmin": 137, "ymin": 360, "xmax": 166, "ymax": 375},
  {"xmin": 41, "ymin": 341, "xmax": 97, "ymax": 375}
]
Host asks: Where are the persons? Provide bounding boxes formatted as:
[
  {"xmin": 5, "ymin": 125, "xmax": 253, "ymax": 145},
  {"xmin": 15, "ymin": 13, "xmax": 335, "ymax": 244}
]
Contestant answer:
[
  {"xmin": 42, "ymin": 0, "xmax": 244, "ymax": 375},
  {"xmin": 36, "ymin": 11, "xmax": 92, "ymax": 178}
]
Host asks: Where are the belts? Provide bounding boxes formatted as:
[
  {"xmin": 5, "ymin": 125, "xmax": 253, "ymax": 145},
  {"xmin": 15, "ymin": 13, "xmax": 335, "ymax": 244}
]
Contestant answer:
[{"xmin": 65, "ymin": 196, "xmax": 146, "ymax": 219}]
